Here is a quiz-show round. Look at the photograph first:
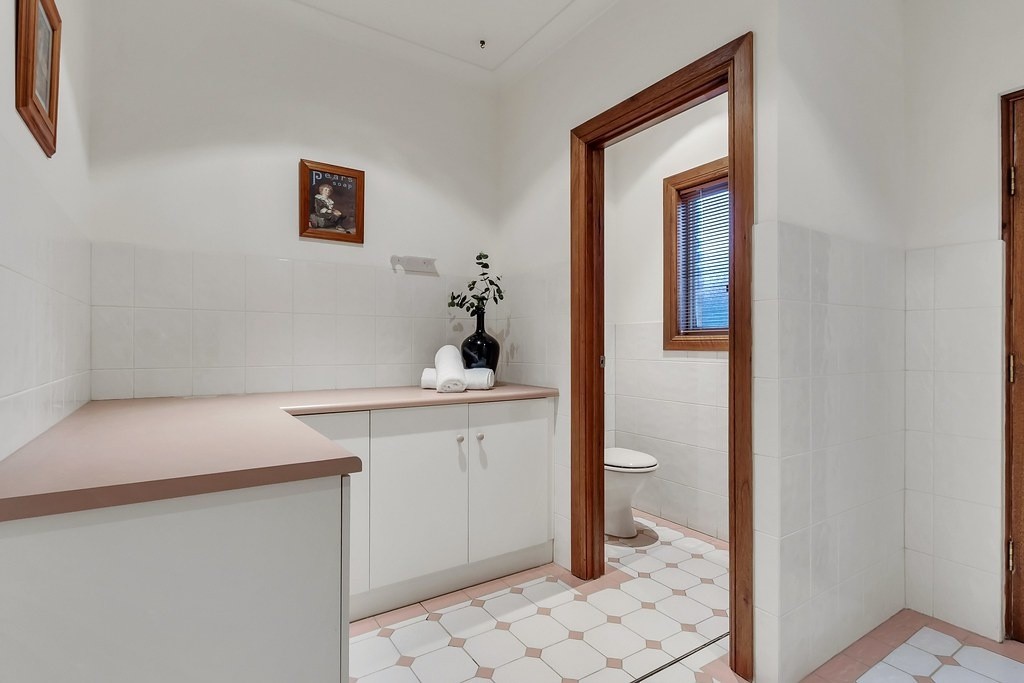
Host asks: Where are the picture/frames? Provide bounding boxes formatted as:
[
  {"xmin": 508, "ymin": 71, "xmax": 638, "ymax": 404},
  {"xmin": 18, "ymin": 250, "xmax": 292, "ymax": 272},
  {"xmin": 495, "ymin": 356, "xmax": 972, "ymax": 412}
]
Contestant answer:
[
  {"xmin": 298, "ymin": 159, "xmax": 365, "ymax": 244},
  {"xmin": 15, "ymin": 0, "xmax": 62, "ymax": 159}
]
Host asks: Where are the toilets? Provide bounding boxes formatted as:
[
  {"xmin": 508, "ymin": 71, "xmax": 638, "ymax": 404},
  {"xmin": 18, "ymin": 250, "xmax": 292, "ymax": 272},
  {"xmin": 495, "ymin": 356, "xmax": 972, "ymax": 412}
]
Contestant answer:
[{"xmin": 604, "ymin": 447, "xmax": 659, "ymax": 538}]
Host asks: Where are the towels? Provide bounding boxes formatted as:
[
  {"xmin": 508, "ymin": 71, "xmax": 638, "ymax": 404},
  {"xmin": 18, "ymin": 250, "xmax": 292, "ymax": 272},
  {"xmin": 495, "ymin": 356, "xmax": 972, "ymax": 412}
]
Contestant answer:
[{"xmin": 420, "ymin": 344, "xmax": 495, "ymax": 393}]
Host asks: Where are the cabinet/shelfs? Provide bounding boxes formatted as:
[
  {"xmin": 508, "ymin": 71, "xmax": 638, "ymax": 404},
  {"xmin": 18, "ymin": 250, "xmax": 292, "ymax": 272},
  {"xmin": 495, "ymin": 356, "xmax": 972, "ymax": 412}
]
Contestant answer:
[
  {"xmin": 283, "ymin": 407, "xmax": 369, "ymax": 626},
  {"xmin": 369, "ymin": 393, "xmax": 555, "ymax": 616}
]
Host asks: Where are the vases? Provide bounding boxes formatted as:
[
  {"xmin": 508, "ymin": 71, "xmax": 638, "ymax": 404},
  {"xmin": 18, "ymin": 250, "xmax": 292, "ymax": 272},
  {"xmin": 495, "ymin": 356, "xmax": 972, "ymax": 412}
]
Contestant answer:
[{"xmin": 460, "ymin": 313, "xmax": 500, "ymax": 375}]
